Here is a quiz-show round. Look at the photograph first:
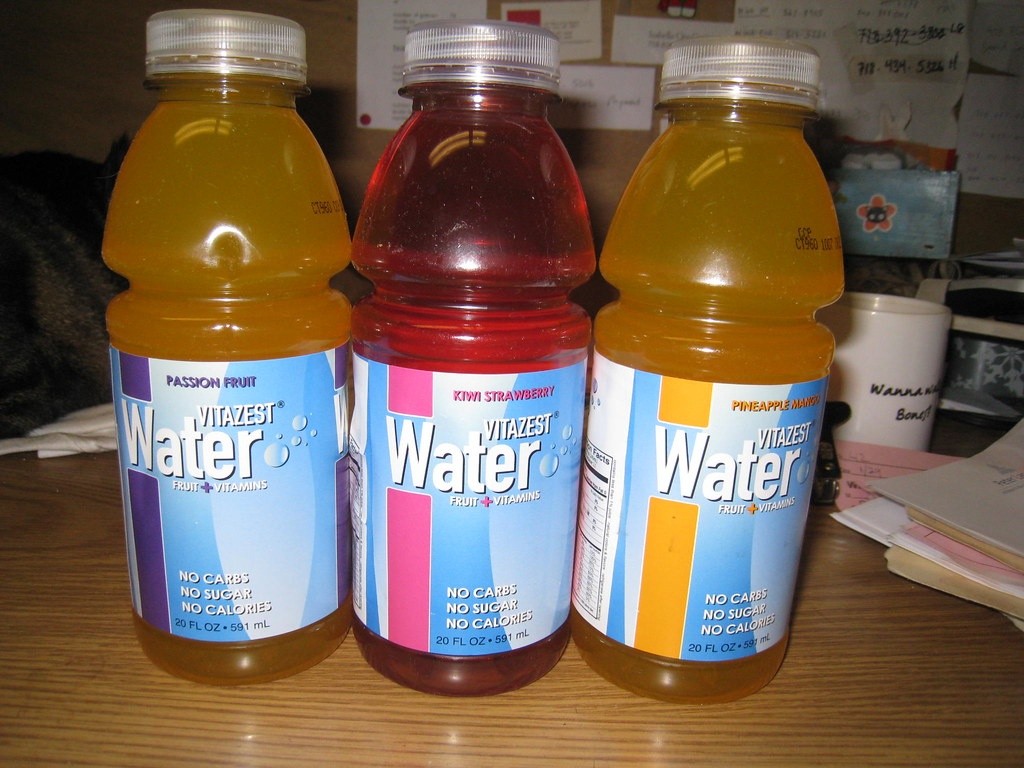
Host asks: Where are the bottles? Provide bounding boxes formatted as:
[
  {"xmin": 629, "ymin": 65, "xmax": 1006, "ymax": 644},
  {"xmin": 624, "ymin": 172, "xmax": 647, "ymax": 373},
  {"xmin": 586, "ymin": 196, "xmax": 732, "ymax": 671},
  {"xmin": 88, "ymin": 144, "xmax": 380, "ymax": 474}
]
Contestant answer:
[
  {"xmin": 572, "ymin": 36, "xmax": 846, "ymax": 705},
  {"xmin": 101, "ymin": 9, "xmax": 352, "ymax": 687},
  {"xmin": 352, "ymin": 18, "xmax": 598, "ymax": 699}
]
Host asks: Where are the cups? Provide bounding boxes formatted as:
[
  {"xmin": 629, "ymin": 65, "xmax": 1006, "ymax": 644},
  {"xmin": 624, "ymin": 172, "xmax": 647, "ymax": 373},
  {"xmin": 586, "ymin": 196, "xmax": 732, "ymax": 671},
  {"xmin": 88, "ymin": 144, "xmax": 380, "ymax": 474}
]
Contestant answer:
[{"xmin": 815, "ymin": 291, "xmax": 952, "ymax": 452}]
